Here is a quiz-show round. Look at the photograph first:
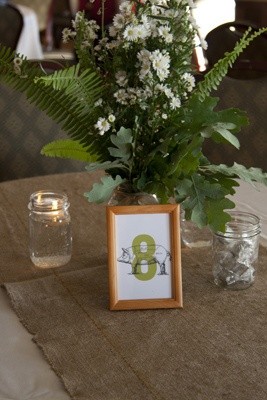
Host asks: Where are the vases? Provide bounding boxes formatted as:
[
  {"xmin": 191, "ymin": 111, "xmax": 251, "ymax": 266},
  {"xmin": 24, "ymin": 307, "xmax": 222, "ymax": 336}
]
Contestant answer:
[{"xmin": 108, "ymin": 182, "xmax": 160, "ymax": 204}]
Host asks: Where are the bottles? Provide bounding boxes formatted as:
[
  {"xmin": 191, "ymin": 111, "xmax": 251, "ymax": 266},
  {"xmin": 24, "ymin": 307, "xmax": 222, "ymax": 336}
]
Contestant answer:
[
  {"xmin": 211, "ymin": 210, "xmax": 262, "ymax": 290},
  {"xmin": 28, "ymin": 189, "xmax": 72, "ymax": 269}
]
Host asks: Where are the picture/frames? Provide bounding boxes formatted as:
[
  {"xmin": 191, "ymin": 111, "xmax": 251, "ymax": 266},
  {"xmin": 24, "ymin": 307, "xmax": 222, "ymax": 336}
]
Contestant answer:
[{"xmin": 105, "ymin": 203, "xmax": 185, "ymax": 311}]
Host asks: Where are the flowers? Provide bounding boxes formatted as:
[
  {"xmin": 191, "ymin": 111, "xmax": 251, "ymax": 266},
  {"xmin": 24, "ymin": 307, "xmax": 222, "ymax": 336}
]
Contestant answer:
[{"xmin": 0, "ymin": 0, "xmax": 267, "ymax": 233}]
